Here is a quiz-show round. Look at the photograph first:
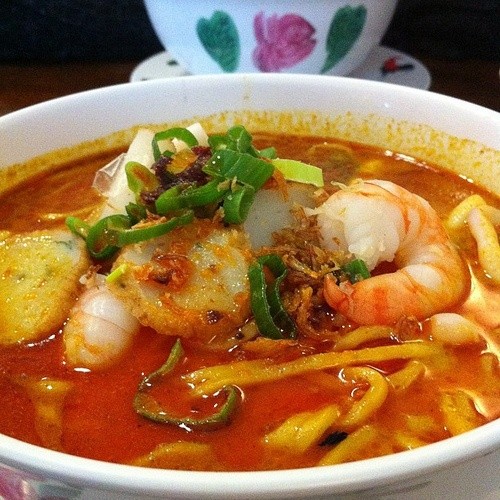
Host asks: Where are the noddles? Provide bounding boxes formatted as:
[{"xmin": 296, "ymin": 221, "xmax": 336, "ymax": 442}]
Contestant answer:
[{"xmin": 123, "ymin": 193, "xmax": 500, "ymax": 474}]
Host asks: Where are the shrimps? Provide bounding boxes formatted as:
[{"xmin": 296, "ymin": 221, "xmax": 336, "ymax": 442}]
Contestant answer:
[{"xmin": 317, "ymin": 177, "xmax": 472, "ymax": 326}]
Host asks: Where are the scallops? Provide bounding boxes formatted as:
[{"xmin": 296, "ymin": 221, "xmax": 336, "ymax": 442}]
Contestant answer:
[
  {"xmin": 109, "ymin": 215, "xmax": 253, "ymax": 336},
  {"xmin": 62, "ymin": 279, "xmax": 139, "ymax": 374}
]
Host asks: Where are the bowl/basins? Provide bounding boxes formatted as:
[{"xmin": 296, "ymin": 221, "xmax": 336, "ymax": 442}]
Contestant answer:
[
  {"xmin": 0, "ymin": 74, "xmax": 500, "ymax": 500},
  {"xmin": 144, "ymin": 1, "xmax": 397, "ymax": 76}
]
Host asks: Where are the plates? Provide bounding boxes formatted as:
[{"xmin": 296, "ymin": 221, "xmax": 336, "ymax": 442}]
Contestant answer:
[{"xmin": 129, "ymin": 46, "xmax": 433, "ymax": 92}]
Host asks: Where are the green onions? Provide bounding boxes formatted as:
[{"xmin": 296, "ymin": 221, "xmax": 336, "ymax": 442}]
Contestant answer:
[{"xmin": 64, "ymin": 122, "xmax": 370, "ymax": 432}]
